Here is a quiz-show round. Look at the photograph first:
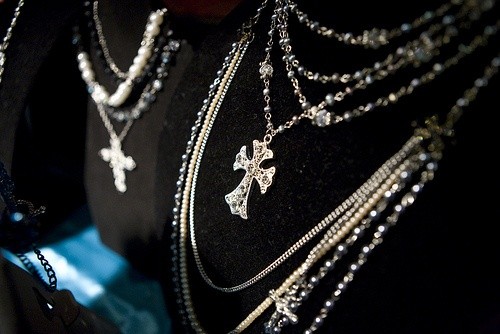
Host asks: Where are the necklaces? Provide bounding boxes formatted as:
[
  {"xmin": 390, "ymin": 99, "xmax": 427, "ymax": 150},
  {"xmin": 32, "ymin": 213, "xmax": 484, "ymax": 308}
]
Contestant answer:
[{"xmin": 0, "ymin": 2, "xmax": 500, "ymax": 334}]
